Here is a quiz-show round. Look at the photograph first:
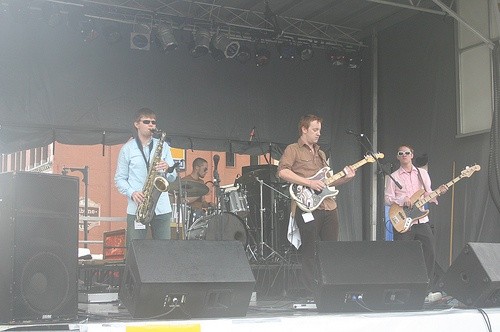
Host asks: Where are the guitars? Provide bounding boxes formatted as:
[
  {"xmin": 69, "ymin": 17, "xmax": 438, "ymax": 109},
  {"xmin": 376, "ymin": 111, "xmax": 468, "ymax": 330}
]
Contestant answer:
[
  {"xmin": 289, "ymin": 153, "xmax": 384, "ymax": 213},
  {"xmin": 389, "ymin": 163, "xmax": 481, "ymax": 233}
]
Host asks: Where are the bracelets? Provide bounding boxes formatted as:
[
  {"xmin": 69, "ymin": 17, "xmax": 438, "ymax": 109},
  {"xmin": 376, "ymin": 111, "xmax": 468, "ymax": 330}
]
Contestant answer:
[{"xmin": 208, "ymin": 202, "xmax": 211, "ymax": 205}]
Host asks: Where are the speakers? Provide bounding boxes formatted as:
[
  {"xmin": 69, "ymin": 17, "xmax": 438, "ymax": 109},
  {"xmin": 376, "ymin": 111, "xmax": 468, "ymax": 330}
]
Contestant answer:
[
  {"xmin": 435, "ymin": 242, "xmax": 500, "ymax": 309},
  {"xmin": 118, "ymin": 239, "xmax": 255, "ymax": 319},
  {"xmin": 0, "ymin": 170, "xmax": 79, "ymax": 323},
  {"xmin": 313, "ymin": 240, "xmax": 430, "ymax": 312}
]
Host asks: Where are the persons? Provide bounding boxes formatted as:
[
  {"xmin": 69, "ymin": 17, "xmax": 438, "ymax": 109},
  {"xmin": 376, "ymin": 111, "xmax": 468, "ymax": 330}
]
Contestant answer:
[
  {"xmin": 384, "ymin": 143, "xmax": 449, "ymax": 303},
  {"xmin": 114, "ymin": 108, "xmax": 177, "ymax": 250},
  {"xmin": 276, "ymin": 114, "xmax": 355, "ymax": 287},
  {"xmin": 175, "ymin": 158, "xmax": 216, "ymax": 217}
]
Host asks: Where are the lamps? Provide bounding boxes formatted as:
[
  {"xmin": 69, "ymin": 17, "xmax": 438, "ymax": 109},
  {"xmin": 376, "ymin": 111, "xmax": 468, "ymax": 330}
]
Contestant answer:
[{"xmin": 130, "ymin": 13, "xmax": 363, "ymax": 70}]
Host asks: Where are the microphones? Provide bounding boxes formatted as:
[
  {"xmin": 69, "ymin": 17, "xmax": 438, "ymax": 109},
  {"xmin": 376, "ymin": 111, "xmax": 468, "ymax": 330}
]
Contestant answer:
[
  {"xmin": 346, "ymin": 129, "xmax": 365, "ymax": 137},
  {"xmin": 233, "ymin": 175, "xmax": 238, "ymax": 187},
  {"xmin": 249, "ymin": 128, "xmax": 254, "ymax": 142}
]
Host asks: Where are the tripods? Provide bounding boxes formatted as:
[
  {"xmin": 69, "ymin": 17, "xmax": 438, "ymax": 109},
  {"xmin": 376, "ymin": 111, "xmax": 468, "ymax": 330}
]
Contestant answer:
[{"xmin": 248, "ymin": 174, "xmax": 290, "ymax": 265}]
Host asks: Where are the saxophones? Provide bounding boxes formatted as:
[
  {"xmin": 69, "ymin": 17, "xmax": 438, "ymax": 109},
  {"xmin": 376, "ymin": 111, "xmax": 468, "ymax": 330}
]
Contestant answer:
[{"xmin": 135, "ymin": 127, "xmax": 170, "ymax": 225}]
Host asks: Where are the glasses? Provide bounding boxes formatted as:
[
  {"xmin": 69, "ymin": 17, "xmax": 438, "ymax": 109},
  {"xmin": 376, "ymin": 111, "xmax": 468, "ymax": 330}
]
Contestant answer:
[
  {"xmin": 139, "ymin": 120, "xmax": 156, "ymax": 124},
  {"xmin": 202, "ymin": 168, "xmax": 208, "ymax": 172},
  {"xmin": 397, "ymin": 151, "xmax": 411, "ymax": 156}
]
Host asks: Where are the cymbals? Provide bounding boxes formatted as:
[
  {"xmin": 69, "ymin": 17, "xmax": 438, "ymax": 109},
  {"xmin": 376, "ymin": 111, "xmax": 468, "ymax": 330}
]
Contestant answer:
[{"xmin": 168, "ymin": 179, "xmax": 210, "ymax": 197}]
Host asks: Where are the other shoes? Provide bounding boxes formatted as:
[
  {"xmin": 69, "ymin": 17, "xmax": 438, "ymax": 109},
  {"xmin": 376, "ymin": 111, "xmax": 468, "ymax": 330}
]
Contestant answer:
[{"xmin": 424, "ymin": 292, "xmax": 441, "ymax": 303}]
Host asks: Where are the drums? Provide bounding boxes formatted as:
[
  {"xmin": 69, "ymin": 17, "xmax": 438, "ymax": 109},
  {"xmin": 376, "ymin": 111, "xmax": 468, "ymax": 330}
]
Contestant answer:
[
  {"xmin": 187, "ymin": 212, "xmax": 248, "ymax": 253},
  {"xmin": 169, "ymin": 203, "xmax": 191, "ymax": 236},
  {"xmin": 223, "ymin": 185, "xmax": 251, "ymax": 218}
]
